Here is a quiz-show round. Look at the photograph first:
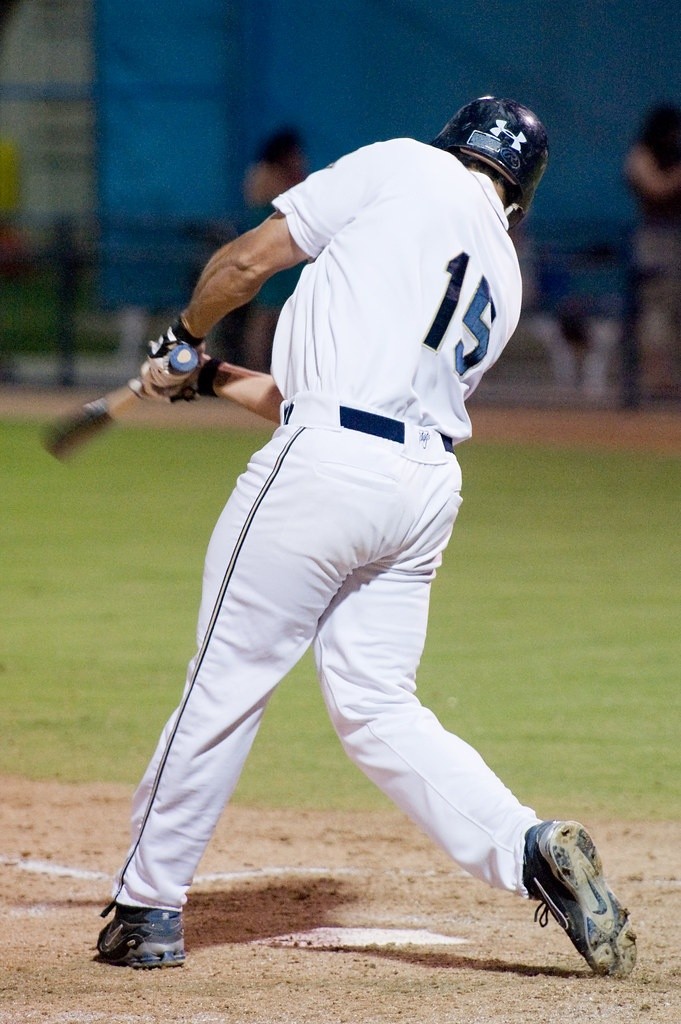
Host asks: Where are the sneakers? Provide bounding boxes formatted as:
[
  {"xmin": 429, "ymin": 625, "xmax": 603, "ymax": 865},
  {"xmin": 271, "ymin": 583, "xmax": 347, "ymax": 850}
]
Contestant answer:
[
  {"xmin": 96, "ymin": 904, "xmax": 186, "ymax": 969},
  {"xmin": 522, "ymin": 819, "xmax": 636, "ymax": 977}
]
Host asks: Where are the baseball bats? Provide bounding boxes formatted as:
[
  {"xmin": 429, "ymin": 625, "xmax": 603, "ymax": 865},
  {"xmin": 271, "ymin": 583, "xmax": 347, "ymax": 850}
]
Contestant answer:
[{"xmin": 36, "ymin": 343, "xmax": 201, "ymax": 465}]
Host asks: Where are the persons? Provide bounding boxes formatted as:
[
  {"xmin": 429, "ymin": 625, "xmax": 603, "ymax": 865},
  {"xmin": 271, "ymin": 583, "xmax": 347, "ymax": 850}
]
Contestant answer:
[
  {"xmin": 239, "ymin": 129, "xmax": 311, "ymax": 378},
  {"xmin": 618, "ymin": 102, "xmax": 681, "ymax": 410},
  {"xmin": 96, "ymin": 94, "xmax": 639, "ymax": 972}
]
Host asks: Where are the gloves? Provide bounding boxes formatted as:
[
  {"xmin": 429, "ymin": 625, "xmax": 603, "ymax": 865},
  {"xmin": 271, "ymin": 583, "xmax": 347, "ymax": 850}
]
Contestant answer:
[{"xmin": 140, "ymin": 314, "xmax": 224, "ymax": 404}]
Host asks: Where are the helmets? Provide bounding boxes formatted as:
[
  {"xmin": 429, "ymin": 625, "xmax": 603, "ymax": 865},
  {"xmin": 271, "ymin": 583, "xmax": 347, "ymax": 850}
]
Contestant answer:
[{"xmin": 430, "ymin": 95, "xmax": 549, "ymax": 230}]
